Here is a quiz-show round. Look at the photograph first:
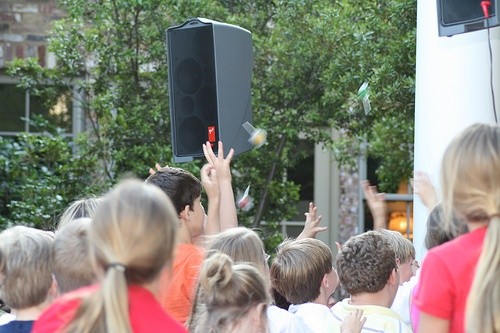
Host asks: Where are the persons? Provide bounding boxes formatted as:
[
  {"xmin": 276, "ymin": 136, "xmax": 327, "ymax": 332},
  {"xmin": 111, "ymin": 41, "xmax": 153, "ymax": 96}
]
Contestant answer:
[
  {"xmin": 201, "ymin": 253, "xmax": 267, "ymax": 333},
  {"xmin": 0, "ymin": 226, "xmax": 58, "ymax": 333},
  {"xmin": 362, "ymin": 170, "xmax": 469, "ymax": 332},
  {"xmin": 326, "ymin": 231, "xmax": 414, "ymax": 333},
  {"xmin": 205, "ymin": 226, "xmax": 313, "ymax": 332},
  {"xmin": 295, "ymin": 202, "xmax": 328, "ymax": 239},
  {"xmin": 409, "ymin": 122, "xmax": 500, "ymax": 333},
  {"xmin": 52, "ymin": 197, "xmax": 104, "ymax": 296},
  {"xmin": 142, "ymin": 140, "xmax": 238, "ymax": 325},
  {"xmin": 270, "ymin": 238, "xmax": 366, "ymax": 333},
  {"xmin": 32, "ymin": 178, "xmax": 191, "ymax": 333}
]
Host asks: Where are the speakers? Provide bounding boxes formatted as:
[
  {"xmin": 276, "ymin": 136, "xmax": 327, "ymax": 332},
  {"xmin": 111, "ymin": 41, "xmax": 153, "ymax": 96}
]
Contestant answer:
[
  {"xmin": 436, "ymin": 0, "xmax": 500, "ymax": 37},
  {"xmin": 166, "ymin": 17, "xmax": 255, "ymax": 164}
]
